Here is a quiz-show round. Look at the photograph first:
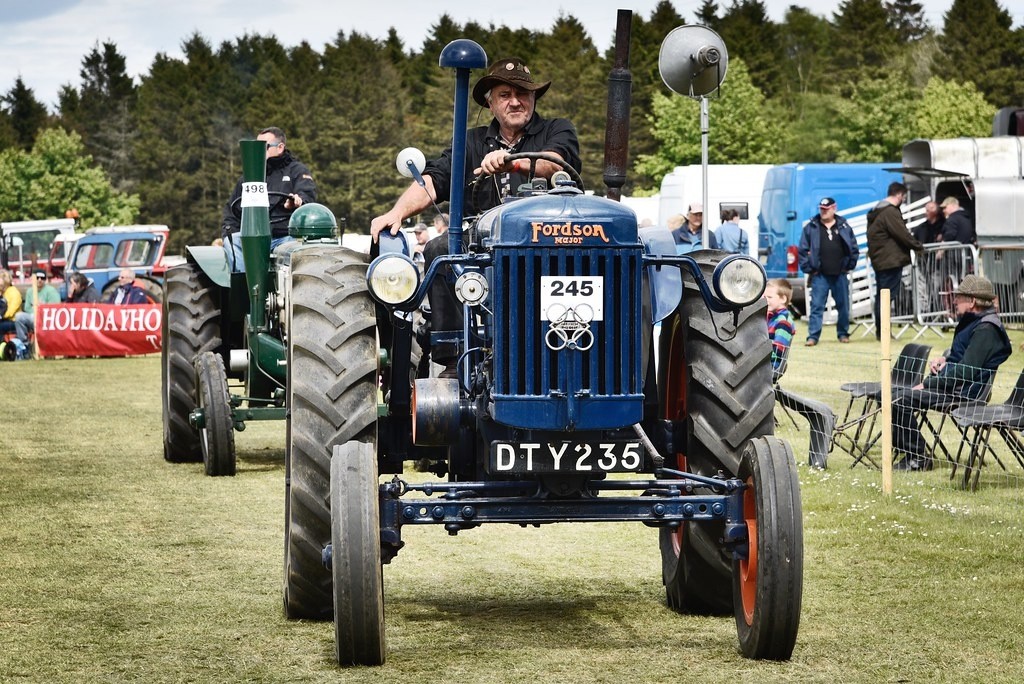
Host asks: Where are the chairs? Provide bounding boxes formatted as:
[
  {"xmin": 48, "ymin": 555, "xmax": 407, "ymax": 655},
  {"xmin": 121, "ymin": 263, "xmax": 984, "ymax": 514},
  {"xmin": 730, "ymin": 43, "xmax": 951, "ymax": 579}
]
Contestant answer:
[{"xmin": 838, "ymin": 344, "xmax": 1024, "ymax": 492}]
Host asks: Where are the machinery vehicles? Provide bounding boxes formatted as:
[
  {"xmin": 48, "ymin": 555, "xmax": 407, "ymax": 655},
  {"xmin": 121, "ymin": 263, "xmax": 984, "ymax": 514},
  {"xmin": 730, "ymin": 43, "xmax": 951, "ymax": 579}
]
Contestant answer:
[
  {"xmin": 277, "ymin": 7, "xmax": 805, "ymax": 670},
  {"xmin": 156, "ymin": 137, "xmax": 372, "ymax": 476}
]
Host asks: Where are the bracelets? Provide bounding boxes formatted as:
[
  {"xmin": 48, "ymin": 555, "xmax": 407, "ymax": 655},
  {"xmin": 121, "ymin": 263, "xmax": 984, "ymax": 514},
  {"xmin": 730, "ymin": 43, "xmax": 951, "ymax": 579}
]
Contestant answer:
[{"xmin": 511, "ymin": 161, "xmax": 520, "ymax": 174}]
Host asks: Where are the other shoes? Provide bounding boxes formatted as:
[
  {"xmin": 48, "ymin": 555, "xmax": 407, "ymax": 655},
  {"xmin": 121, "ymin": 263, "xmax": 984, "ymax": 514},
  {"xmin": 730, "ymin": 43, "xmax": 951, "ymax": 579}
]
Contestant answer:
[
  {"xmin": 805, "ymin": 339, "xmax": 815, "ymax": 346},
  {"xmin": 839, "ymin": 336, "xmax": 849, "ymax": 343},
  {"xmin": 893, "ymin": 454, "xmax": 932, "ymax": 471}
]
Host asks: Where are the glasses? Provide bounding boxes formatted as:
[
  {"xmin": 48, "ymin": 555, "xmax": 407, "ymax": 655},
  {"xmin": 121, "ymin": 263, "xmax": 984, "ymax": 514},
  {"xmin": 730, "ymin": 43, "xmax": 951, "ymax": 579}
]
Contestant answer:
[
  {"xmin": 37, "ymin": 276, "xmax": 46, "ymax": 280},
  {"xmin": 267, "ymin": 142, "xmax": 280, "ymax": 150}
]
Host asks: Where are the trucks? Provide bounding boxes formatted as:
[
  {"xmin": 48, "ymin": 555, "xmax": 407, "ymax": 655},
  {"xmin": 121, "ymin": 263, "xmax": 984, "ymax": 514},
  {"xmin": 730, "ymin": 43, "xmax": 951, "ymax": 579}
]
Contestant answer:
[
  {"xmin": 0, "ymin": 207, "xmax": 188, "ymax": 359},
  {"xmin": 583, "ymin": 107, "xmax": 1024, "ymax": 324}
]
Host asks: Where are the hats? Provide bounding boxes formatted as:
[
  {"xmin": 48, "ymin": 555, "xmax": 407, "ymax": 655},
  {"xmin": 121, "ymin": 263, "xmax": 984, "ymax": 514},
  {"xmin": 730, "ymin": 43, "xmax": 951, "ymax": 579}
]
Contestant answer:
[
  {"xmin": 939, "ymin": 275, "xmax": 994, "ymax": 296},
  {"xmin": 940, "ymin": 197, "xmax": 959, "ymax": 208},
  {"xmin": 414, "ymin": 221, "xmax": 427, "ymax": 233},
  {"xmin": 818, "ymin": 198, "xmax": 836, "ymax": 208},
  {"xmin": 473, "ymin": 58, "xmax": 551, "ymax": 108},
  {"xmin": 688, "ymin": 202, "xmax": 702, "ymax": 214}
]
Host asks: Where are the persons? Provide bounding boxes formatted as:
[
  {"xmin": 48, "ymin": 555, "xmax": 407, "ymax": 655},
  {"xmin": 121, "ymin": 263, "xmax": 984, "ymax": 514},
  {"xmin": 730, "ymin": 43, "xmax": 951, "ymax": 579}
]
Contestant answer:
[
  {"xmin": 867, "ymin": 182, "xmax": 924, "ymax": 341},
  {"xmin": 714, "ymin": 209, "xmax": 749, "ymax": 255},
  {"xmin": 891, "ymin": 273, "xmax": 1012, "ymax": 471},
  {"xmin": 912, "ymin": 202, "xmax": 946, "ymax": 322},
  {"xmin": 763, "ymin": 279, "xmax": 796, "ymax": 384},
  {"xmin": 433, "ymin": 213, "xmax": 449, "ymax": 234},
  {"xmin": 798, "ymin": 197, "xmax": 859, "ymax": 346},
  {"xmin": 0, "ymin": 269, "xmax": 23, "ymax": 342},
  {"xmin": 67, "ymin": 272, "xmax": 101, "ymax": 359},
  {"xmin": 406, "ymin": 223, "xmax": 431, "ymax": 283},
  {"xmin": 370, "ymin": 57, "xmax": 583, "ymax": 378},
  {"xmin": 15, "ymin": 269, "xmax": 61, "ymax": 360},
  {"xmin": 98, "ymin": 269, "xmax": 149, "ymax": 358},
  {"xmin": 935, "ymin": 196, "xmax": 973, "ymax": 332},
  {"xmin": 221, "ymin": 127, "xmax": 318, "ymax": 273},
  {"xmin": 671, "ymin": 203, "xmax": 718, "ymax": 249}
]
